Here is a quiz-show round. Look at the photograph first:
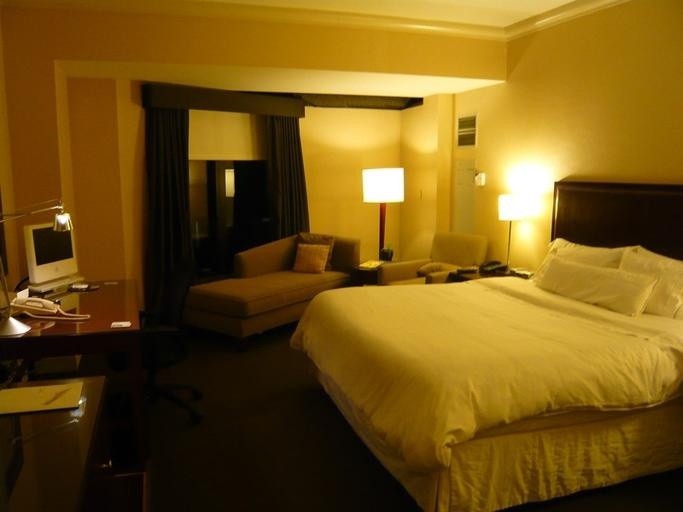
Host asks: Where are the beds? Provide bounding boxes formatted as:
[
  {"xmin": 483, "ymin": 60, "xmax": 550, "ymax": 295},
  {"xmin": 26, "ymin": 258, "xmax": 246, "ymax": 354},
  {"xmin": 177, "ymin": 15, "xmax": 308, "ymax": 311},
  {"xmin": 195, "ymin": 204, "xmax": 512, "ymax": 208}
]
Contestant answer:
[{"xmin": 309, "ymin": 179, "xmax": 683, "ymax": 512}]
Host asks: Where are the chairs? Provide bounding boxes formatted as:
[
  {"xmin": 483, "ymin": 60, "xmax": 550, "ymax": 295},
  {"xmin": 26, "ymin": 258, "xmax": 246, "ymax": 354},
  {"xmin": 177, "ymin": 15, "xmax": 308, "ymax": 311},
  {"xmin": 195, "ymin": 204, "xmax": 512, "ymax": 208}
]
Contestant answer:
[
  {"xmin": 106, "ymin": 264, "xmax": 204, "ymax": 423},
  {"xmin": 378, "ymin": 230, "xmax": 488, "ymax": 285},
  {"xmin": 187, "ymin": 232, "xmax": 361, "ymax": 340}
]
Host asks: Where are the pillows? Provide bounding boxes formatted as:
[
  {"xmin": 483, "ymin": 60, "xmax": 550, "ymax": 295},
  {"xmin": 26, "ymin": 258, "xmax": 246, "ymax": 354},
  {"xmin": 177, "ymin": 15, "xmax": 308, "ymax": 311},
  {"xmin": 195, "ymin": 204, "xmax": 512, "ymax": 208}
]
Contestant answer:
[
  {"xmin": 530, "ymin": 236, "xmax": 681, "ymax": 321},
  {"xmin": 293, "ymin": 230, "xmax": 335, "ymax": 275}
]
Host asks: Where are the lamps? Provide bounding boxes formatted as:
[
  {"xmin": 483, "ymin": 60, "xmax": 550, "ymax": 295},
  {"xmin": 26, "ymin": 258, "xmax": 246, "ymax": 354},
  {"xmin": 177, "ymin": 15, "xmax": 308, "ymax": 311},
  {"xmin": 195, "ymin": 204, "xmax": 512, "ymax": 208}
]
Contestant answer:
[
  {"xmin": 361, "ymin": 167, "xmax": 405, "ymax": 259},
  {"xmin": 0, "ymin": 198, "xmax": 74, "ymax": 338}
]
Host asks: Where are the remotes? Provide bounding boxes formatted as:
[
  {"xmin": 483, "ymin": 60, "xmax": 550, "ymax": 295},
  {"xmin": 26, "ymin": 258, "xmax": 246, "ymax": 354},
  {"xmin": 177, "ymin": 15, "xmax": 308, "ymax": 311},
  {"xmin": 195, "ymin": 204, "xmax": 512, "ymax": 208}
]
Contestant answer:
[{"xmin": 457, "ymin": 267, "xmax": 478, "ymax": 272}]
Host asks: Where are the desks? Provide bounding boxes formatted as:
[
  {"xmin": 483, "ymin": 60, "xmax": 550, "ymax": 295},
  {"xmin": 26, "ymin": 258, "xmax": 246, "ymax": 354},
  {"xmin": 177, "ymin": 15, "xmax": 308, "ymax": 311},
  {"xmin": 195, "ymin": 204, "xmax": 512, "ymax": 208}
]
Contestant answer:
[{"xmin": 0, "ymin": 275, "xmax": 142, "ymax": 509}]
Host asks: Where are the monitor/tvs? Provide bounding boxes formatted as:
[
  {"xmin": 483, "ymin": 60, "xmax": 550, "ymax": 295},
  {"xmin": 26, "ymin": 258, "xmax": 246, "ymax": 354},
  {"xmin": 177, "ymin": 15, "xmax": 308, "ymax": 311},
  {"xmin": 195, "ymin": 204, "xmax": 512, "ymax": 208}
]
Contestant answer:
[{"xmin": 23, "ymin": 222, "xmax": 85, "ymax": 293}]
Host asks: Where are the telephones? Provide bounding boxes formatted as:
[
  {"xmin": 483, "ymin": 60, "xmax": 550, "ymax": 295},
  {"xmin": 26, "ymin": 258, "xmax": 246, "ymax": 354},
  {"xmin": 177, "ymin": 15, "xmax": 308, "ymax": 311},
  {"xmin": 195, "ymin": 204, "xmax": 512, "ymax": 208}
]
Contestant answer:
[
  {"xmin": 10, "ymin": 289, "xmax": 60, "ymax": 316},
  {"xmin": 479, "ymin": 260, "xmax": 507, "ymax": 274}
]
